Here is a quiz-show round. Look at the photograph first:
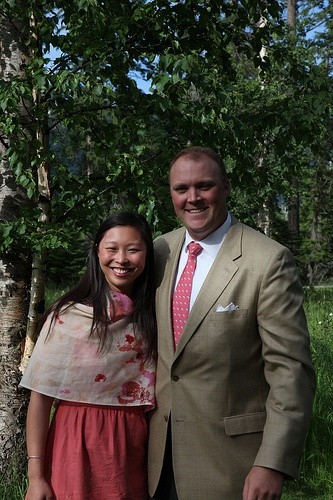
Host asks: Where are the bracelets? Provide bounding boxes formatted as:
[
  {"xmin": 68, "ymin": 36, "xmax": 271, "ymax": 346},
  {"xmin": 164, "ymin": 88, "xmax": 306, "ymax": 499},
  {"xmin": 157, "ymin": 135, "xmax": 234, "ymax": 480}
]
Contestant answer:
[{"xmin": 27, "ymin": 455, "xmax": 44, "ymax": 460}]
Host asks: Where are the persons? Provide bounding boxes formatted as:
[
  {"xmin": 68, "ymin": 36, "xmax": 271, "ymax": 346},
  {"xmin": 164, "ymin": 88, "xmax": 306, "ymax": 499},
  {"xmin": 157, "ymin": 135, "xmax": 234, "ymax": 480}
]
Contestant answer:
[
  {"xmin": 145, "ymin": 152, "xmax": 314, "ymax": 500},
  {"xmin": 15, "ymin": 212, "xmax": 163, "ymax": 500}
]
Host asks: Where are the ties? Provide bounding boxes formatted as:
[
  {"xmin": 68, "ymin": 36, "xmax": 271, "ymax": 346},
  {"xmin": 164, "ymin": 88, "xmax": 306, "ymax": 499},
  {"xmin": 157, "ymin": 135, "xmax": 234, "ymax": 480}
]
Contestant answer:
[{"xmin": 173, "ymin": 242, "xmax": 204, "ymax": 351}]
